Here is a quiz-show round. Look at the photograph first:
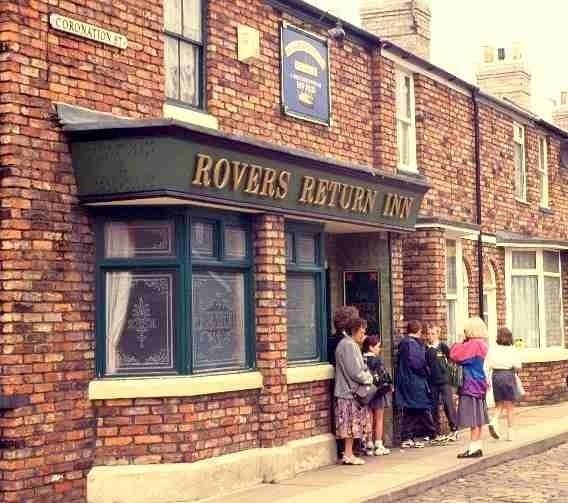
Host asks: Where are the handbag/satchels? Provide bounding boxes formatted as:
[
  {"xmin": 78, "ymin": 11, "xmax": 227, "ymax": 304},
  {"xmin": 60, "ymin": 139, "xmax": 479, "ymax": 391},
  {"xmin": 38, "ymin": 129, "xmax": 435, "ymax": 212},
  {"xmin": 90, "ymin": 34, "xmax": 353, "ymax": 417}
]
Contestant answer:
[
  {"xmin": 513, "ymin": 375, "xmax": 526, "ymax": 402},
  {"xmin": 353, "ymin": 383, "xmax": 378, "ymax": 406}
]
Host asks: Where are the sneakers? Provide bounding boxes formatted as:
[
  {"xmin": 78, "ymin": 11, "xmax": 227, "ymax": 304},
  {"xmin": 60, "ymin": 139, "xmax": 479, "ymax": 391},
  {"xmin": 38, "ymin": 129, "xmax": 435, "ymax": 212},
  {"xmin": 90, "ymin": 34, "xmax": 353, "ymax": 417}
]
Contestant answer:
[{"xmin": 340, "ymin": 418, "xmax": 515, "ymax": 465}]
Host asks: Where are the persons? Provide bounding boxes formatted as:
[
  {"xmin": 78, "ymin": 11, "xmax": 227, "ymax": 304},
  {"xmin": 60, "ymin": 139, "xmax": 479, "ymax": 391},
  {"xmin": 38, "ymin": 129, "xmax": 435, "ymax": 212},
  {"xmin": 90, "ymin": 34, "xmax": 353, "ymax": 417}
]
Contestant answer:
[
  {"xmin": 395, "ymin": 319, "xmax": 458, "ymax": 449},
  {"xmin": 448, "ymin": 316, "xmax": 489, "ymax": 458},
  {"xmin": 327, "ymin": 305, "xmax": 395, "ymax": 465},
  {"xmin": 485, "ymin": 327, "xmax": 522, "ymax": 441}
]
